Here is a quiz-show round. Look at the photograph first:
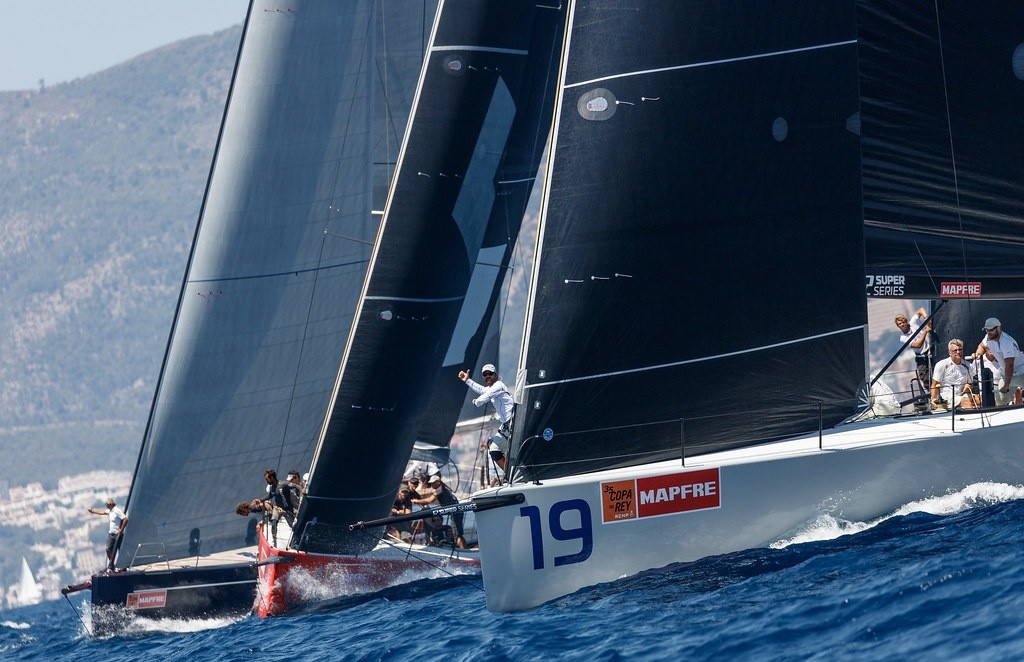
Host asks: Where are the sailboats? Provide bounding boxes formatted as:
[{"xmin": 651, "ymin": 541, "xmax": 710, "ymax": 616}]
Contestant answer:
[
  {"xmin": 250, "ymin": 0, "xmax": 568, "ymax": 621},
  {"xmin": 61, "ymin": 0, "xmax": 443, "ymax": 635},
  {"xmin": 348, "ymin": 1, "xmax": 1024, "ymax": 614}
]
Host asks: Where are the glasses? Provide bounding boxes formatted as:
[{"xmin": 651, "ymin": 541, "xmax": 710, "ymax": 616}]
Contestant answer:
[
  {"xmin": 482, "ymin": 372, "xmax": 494, "ymax": 376},
  {"xmin": 985, "ymin": 328, "xmax": 995, "ymax": 332}
]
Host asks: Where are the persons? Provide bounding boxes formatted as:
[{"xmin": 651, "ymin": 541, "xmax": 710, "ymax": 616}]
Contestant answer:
[
  {"xmin": 236, "ymin": 470, "xmax": 309, "ymax": 539},
  {"xmin": 931, "ymin": 339, "xmax": 973, "ymax": 410},
  {"xmin": 459, "ymin": 363, "xmax": 514, "ymax": 484},
  {"xmin": 88, "ymin": 498, "xmax": 128, "ymax": 573},
  {"xmin": 896, "ymin": 307, "xmax": 931, "ymax": 392},
  {"xmin": 388, "ymin": 460, "xmax": 466, "ymax": 549},
  {"xmin": 972, "ymin": 318, "xmax": 1024, "ymax": 405}
]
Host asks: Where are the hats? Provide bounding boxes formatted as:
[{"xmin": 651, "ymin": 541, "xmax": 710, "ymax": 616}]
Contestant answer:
[
  {"xmin": 286, "ymin": 473, "xmax": 296, "ymax": 481},
  {"xmin": 482, "ymin": 363, "xmax": 497, "ymax": 374},
  {"xmin": 982, "ymin": 318, "xmax": 1001, "ymax": 331},
  {"xmin": 409, "ymin": 477, "xmax": 420, "ymax": 484},
  {"xmin": 428, "ymin": 475, "xmax": 441, "ymax": 483},
  {"xmin": 400, "ymin": 486, "xmax": 410, "ymax": 492}
]
